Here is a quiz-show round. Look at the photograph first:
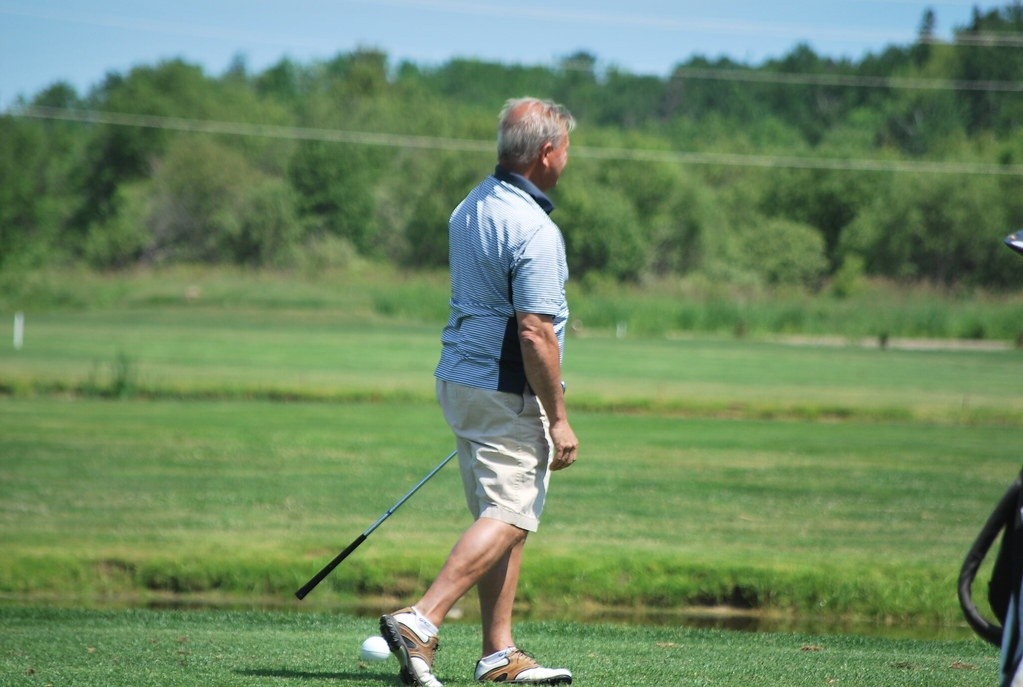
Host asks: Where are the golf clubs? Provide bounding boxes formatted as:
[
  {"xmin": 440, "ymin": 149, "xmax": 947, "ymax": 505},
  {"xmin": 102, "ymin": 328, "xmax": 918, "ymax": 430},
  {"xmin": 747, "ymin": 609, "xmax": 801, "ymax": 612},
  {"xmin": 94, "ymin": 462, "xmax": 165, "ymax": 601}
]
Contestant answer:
[{"xmin": 293, "ymin": 379, "xmax": 567, "ymax": 602}]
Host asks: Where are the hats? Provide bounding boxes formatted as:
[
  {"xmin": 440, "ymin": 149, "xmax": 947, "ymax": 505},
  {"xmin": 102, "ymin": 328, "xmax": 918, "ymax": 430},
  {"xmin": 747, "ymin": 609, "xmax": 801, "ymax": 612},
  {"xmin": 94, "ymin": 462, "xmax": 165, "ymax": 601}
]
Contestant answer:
[{"xmin": 1004, "ymin": 228, "xmax": 1023, "ymax": 249}]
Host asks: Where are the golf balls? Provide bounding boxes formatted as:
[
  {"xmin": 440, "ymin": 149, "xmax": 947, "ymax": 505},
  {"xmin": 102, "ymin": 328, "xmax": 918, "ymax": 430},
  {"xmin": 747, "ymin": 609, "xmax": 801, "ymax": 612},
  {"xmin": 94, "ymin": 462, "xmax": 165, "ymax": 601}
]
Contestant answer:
[{"xmin": 360, "ymin": 635, "xmax": 392, "ymax": 662}]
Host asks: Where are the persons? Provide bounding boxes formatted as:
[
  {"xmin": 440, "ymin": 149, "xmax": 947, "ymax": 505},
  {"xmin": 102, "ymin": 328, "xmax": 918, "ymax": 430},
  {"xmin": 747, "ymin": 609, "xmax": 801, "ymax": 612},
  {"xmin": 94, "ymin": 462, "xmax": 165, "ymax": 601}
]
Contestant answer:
[{"xmin": 377, "ymin": 95, "xmax": 580, "ymax": 686}]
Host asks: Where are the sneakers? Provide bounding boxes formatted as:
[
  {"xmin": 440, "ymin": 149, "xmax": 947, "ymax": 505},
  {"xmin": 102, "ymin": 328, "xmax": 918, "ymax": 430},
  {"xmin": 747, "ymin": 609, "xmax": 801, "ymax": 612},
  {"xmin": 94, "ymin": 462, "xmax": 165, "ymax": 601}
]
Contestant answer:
[
  {"xmin": 379, "ymin": 606, "xmax": 443, "ymax": 687},
  {"xmin": 474, "ymin": 647, "xmax": 573, "ymax": 685}
]
